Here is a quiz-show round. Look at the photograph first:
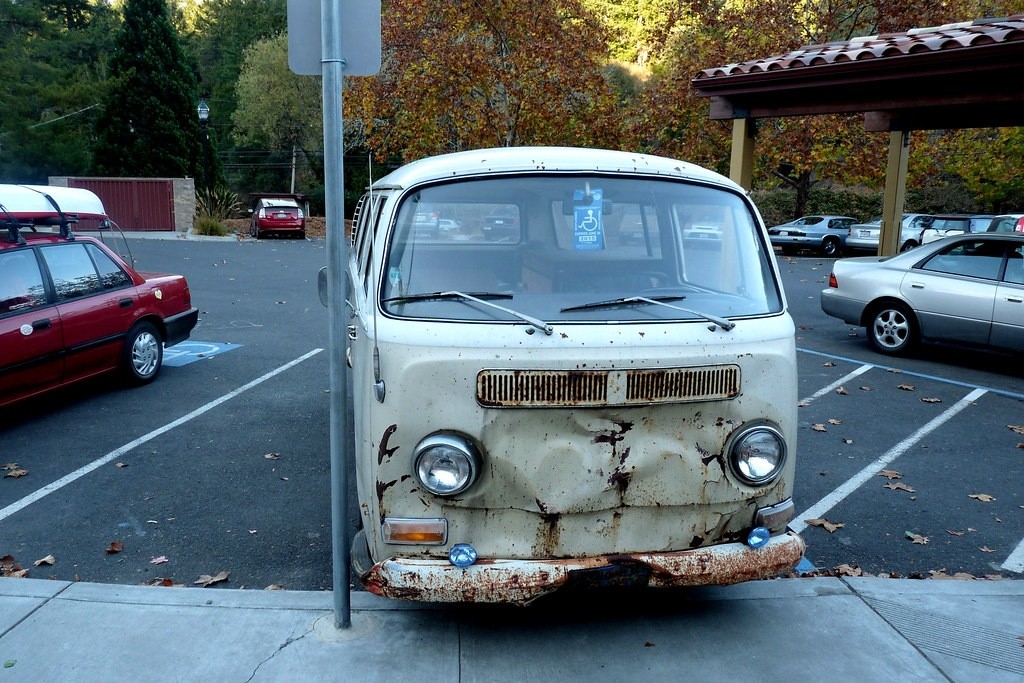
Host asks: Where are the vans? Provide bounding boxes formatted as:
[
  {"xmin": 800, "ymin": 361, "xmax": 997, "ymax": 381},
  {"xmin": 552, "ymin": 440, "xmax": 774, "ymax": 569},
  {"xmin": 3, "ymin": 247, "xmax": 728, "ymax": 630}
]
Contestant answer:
[{"xmin": 315, "ymin": 146, "xmax": 809, "ymax": 609}]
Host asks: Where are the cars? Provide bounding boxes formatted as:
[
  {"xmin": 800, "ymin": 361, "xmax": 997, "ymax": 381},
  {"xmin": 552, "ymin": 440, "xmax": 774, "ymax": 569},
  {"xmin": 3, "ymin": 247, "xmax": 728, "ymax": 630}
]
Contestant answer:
[
  {"xmin": 845, "ymin": 213, "xmax": 931, "ymax": 254},
  {"xmin": 820, "ymin": 230, "xmax": 1024, "ymax": 359},
  {"xmin": 479, "ymin": 203, "xmax": 520, "ymax": 242},
  {"xmin": 766, "ymin": 215, "xmax": 863, "ymax": 258},
  {"xmin": 248, "ymin": 192, "xmax": 307, "ymax": 240},
  {"xmin": 618, "ymin": 211, "xmax": 661, "ymax": 248},
  {"xmin": 410, "ymin": 209, "xmax": 441, "ymax": 240},
  {"xmin": 0, "ymin": 183, "xmax": 200, "ymax": 411},
  {"xmin": 985, "ymin": 214, "xmax": 1024, "ymax": 255},
  {"xmin": 919, "ymin": 214, "xmax": 996, "ymax": 253},
  {"xmin": 439, "ymin": 218, "xmax": 466, "ymax": 237},
  {"xmin": 682, "ymin": 218, "xmax": 724, "ymax": 246}
]
